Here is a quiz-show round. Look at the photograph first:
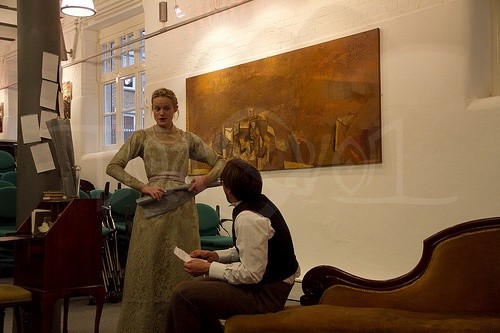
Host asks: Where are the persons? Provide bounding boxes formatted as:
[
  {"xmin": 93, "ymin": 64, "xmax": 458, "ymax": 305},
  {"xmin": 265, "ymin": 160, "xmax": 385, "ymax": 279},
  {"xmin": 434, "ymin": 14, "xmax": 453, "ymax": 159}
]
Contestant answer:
[
  {"xmin": 104, "ymin": 88, "xmax": 227, "ymax": 333},
  {"xmin": 165, "ymin": 158, "xmax": 301, "ymax": 333}
]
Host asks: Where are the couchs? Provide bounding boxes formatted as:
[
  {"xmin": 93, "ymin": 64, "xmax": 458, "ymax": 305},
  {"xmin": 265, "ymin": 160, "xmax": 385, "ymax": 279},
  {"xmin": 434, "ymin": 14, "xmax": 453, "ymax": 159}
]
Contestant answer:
[{"xmin": 222, "ymin": 216, "xmax": 500, "ymax": 333}]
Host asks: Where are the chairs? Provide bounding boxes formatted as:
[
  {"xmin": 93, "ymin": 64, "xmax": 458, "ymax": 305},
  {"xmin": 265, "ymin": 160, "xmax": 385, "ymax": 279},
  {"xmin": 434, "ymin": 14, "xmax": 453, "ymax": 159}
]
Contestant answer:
[{"xmin": 0, "ymin": 149, "xmax": 234, "ymax": 333}]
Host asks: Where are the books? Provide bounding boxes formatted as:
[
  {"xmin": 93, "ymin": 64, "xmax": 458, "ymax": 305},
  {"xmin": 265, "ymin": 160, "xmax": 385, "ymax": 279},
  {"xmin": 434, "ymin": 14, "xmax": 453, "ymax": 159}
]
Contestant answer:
[{"xmin": 42, "ymin": 191, "xmax": 64, "ymax": 201}]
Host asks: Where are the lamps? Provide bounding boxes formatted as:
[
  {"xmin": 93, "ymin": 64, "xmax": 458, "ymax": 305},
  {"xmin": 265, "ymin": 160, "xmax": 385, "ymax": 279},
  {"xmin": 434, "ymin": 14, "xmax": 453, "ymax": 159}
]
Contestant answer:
[{"xmin": 60, "ymin": 0, "xmax": 97, "ymax": 17}]
